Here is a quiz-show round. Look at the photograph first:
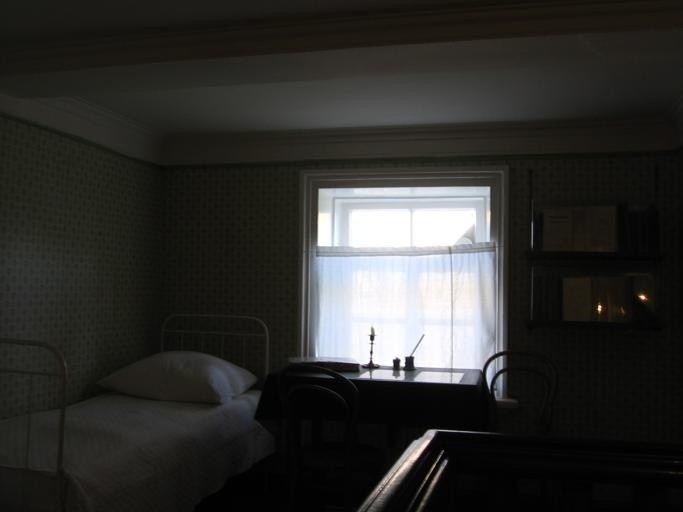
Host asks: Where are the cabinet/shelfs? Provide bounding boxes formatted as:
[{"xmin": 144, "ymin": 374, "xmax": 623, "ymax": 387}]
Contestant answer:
[{"xmin": 526, "ymin": 201, "xmax": 665, "ymax": 332}]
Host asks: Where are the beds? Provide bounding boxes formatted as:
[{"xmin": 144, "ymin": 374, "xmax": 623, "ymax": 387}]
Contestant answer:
[{"xmin": 0, "ymin": 312, "xmax": 275, "ymax": 512}]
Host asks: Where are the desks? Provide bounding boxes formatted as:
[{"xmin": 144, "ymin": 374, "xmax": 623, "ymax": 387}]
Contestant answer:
[{"xmin": 254, "ymin": 362, "xmax": 503, "ymax": 511}]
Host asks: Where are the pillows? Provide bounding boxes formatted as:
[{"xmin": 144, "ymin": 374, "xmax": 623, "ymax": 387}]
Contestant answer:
[{"xmin": 95, "ymin": 350, "xmax": 259, "ymax": 405}]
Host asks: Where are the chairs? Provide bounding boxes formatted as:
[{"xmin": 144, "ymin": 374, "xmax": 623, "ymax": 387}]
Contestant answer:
[
  {"xmin": 271, "ymin": 362, "xmax": 384, "ymax": 512},
  {"xmin": 482, "ymin": 352, "xmax": 558, "ymax": 511}
]
